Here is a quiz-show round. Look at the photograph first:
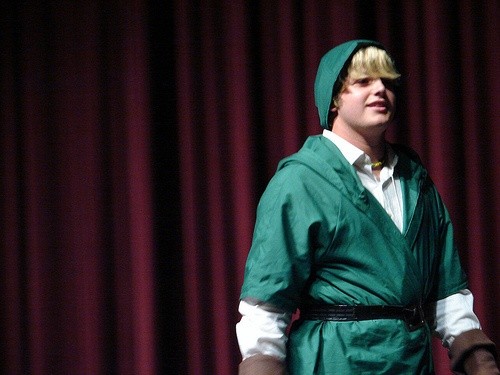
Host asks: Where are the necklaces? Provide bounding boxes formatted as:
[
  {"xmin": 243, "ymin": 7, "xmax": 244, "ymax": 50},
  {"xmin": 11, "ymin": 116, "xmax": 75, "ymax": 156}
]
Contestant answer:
[{"xmin": 371, "ymin": 155, "xmax": 387, "ymax": 169}]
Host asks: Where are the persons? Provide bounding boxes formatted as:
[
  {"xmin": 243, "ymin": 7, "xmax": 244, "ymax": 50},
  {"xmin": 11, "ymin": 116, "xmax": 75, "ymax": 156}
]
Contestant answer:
[{"xmin": 236, "ymin": 40, "xmax": 500, "ymax": 374}]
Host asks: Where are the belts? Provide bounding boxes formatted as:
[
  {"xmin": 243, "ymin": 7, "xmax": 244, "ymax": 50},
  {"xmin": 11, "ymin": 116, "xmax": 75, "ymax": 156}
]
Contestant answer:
[{"xmin": 302, "ymin": 304, "xmax": 431, "ymax": 334}]
